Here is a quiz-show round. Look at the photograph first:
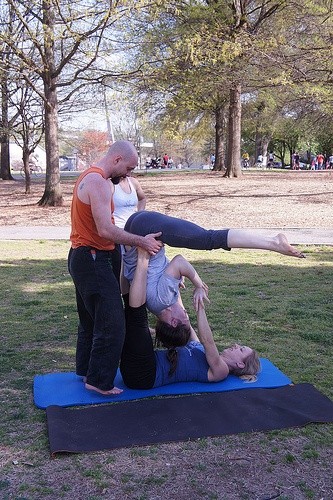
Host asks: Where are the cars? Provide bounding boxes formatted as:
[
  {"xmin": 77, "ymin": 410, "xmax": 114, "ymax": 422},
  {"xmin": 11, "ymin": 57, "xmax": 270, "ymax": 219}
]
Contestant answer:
[{"xmin": 273, "ymin": 161, "xmax": 280, "ymax": 169}]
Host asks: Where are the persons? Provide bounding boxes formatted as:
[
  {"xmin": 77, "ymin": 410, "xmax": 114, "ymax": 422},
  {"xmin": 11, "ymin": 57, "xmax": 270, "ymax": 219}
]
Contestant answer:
[
  {"xmin": 111, "ymin": 173, "xmax": 149, "ymax": 233},
  {"xmin": 120, "ymin": 209, "xmax": 306, "ymax": 379},
  {"xmin": 144, "ymin": 149, "xmax": 333, "ymax": 170},
  {"xmin": 67, "ymin": 139, "xmax": 166, "ymax": 396},
  {"xmin": 115, "ymin": 232, "xmax": 261, "ymax": 390}
]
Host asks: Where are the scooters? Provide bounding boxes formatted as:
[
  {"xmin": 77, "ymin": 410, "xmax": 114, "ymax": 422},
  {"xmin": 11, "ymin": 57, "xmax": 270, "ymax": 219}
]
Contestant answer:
[{"xmin": 152, "ymin": 157, "xmax": 161, "ymax": 167}]
